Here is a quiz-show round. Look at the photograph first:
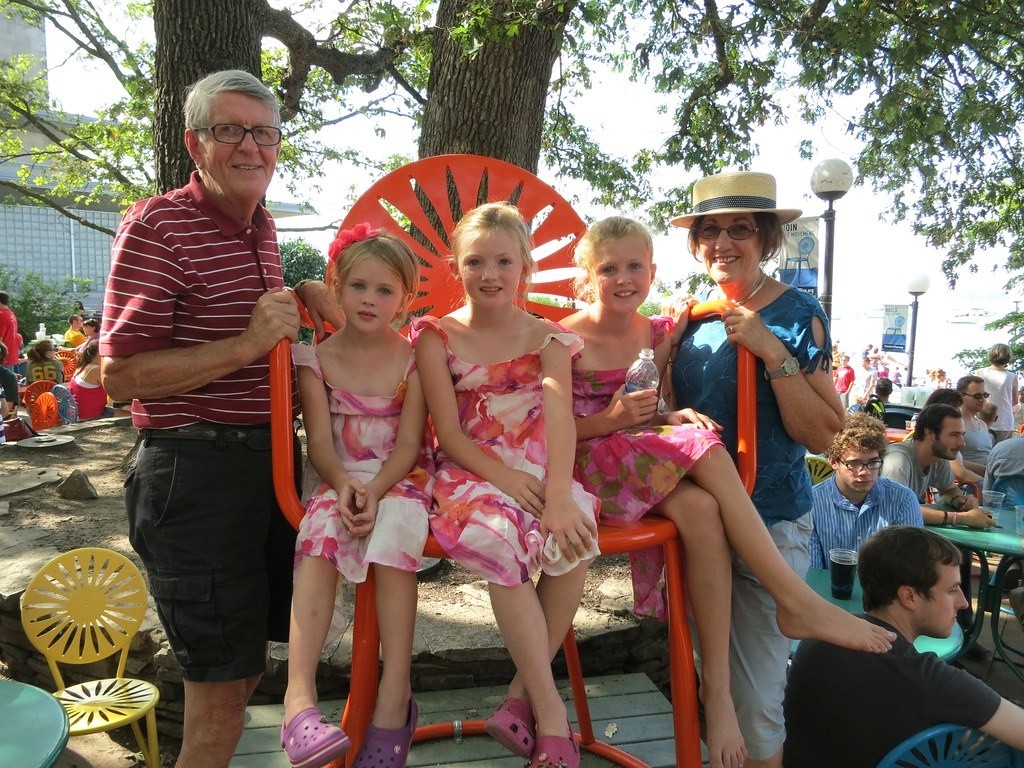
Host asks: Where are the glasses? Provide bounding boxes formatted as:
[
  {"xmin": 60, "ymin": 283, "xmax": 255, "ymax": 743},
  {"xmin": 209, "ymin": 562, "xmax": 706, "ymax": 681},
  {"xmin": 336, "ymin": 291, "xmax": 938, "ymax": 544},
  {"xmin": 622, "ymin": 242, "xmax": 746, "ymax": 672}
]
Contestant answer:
[
  {"xmin": 193, "ymin": 123, "xmax": 283, "ymax": 146},
  {"xmin": 965, "ymin": 392, "xmax": 990, "ymax": 399},
  {"xmin": 837, "ymin": 456, "xmax": 883, "ymax": 470},
  {"xmin": 694, "ymin": 224, "xmax": 760, "ymax": 240}
]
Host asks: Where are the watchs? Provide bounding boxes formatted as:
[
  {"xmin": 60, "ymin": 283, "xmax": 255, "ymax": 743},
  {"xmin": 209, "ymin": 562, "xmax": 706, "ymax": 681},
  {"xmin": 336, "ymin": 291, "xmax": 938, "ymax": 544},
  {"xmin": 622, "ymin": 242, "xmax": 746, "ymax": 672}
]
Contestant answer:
[{"xmin": 764, "ymin": 356, "xmax": 800, "ymax": 380}]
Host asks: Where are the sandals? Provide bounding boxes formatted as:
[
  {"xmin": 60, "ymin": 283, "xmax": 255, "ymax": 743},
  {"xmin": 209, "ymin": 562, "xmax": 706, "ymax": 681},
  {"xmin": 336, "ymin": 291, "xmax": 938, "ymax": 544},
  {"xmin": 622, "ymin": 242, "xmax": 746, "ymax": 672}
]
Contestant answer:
[
  {"xmin": 526, "ymin": 718, "xmax": 581, "ymax": 768},
  {"xmin": 351, "ymin": 695, "xmax": 418, "ymax": 768},
  {"xmin": 280, "ymin": 707, "xmax": 352, "ymax": 768},
  {"xmin": 484, "ymin": 699, "xmax": 536, "ymax": 759}
]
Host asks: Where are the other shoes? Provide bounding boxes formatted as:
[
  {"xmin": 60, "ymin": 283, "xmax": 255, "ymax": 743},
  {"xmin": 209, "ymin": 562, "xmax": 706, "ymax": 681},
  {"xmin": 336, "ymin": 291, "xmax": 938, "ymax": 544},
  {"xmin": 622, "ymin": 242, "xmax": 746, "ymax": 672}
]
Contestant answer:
[{"xmin": 962, "ymin": 639, "xmax": 991, "ymax": 659}]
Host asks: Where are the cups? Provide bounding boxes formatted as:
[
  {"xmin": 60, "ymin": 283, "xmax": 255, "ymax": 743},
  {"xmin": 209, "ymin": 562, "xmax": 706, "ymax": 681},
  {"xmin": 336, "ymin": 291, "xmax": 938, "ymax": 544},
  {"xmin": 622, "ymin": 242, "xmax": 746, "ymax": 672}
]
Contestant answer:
[
  {"xmin": 905, "ymin": 421, "xmax": 915, "ymax": 434},
  {"xmin": 52, "ymin": 333, "xmax": 63, "ymax": 342},
  {"xmin": 828, "ymin": 547, "xmax": 859, "ymax": 599},
  {"xmin": 1015, "ymin": 505, "xmax": 1024, "ymax": 536},
  {"xmin": 36, "ymin": 332, "xmax": 45, "ymax": 339},
  {"xmin": 982, "ymin": 490, "xmax": 1005, "ymax": 519}
]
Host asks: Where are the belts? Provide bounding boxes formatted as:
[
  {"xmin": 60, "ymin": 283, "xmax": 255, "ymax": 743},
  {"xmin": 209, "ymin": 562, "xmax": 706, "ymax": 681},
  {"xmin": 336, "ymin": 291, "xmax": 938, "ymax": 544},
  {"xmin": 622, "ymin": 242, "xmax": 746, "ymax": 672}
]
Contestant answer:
[{"xmin": 150, "ymin": 423, "xmax": 270, "ymax": 442}]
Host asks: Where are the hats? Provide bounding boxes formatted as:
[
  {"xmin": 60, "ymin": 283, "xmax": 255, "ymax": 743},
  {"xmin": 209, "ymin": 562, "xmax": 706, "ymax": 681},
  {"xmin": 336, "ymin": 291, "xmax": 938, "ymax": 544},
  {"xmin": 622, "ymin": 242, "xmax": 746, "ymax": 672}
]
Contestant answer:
[{"xmin": 671, "ymin": 173, "xmax": 803, "ymax": 225}]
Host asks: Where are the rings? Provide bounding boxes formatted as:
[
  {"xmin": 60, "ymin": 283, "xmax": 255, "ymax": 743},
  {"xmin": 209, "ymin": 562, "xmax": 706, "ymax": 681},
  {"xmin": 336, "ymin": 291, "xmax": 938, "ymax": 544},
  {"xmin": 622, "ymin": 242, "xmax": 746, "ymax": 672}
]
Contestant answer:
[{"xmin": 728, "ymin": 325, "xmax": 734, "ymax": 333}]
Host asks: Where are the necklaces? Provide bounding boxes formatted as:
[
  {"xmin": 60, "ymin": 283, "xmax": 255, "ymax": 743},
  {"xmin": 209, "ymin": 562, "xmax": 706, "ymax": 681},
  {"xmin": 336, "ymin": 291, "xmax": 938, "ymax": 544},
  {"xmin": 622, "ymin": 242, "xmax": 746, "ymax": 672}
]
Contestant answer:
[{"xmin": 734, "ymin": 273, "xmax": 767, "ymax": 307}]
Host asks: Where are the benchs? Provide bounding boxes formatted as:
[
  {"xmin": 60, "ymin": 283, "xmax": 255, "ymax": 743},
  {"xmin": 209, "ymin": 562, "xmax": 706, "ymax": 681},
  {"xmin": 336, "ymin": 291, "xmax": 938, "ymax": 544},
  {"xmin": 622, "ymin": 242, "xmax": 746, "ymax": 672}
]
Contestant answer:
[{"xmin": 267, "ymin": 151, "xmax": 760, "ymax": 768}]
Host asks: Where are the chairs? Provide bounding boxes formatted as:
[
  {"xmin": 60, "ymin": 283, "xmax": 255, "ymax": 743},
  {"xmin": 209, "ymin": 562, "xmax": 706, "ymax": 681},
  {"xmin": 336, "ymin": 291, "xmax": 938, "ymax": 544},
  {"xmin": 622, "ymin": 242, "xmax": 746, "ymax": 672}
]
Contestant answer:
[
  {"xmin": 806, "ymin": 457, "xmax": 834, "ymax": 487},
  {"xmin": 925, "ymin": 480, "xmax": 979, "ymax": 504},
  {"xmin": 24, "ymin": 350, "xmax": 79, "ymax": 430},
  {"xmin": 22, "ymin": 547, "xmax": 161, "ymax": 768},
  {"xmin": 876, "ymin": 722, "xmax": 1024, "ymax": 768}
]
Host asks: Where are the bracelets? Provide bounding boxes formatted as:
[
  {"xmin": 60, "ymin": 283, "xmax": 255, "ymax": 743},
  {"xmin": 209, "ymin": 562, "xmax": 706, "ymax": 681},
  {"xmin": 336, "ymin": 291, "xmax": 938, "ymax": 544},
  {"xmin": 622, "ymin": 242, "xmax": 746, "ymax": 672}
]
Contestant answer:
[
  {"xmin": 943, "ymin": 511, "xmax": 948, "ymax": 525},
  {"xmin": 950, "ymin": 495, "xmax": 963, "ymax": 507},
  {"xmin": 952, "ymin": 511, "xmax": 956, "ymax": 527},
  {"xmin": 293, "ymin": 277, "xmax": 313, "ymax": 299}
]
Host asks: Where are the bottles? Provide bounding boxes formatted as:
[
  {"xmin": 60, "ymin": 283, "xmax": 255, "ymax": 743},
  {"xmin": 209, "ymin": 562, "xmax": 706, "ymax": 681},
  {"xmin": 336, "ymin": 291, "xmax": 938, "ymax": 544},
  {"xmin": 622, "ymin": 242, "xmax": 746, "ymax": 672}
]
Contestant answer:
[
  {"xmin": 623, "ymin": 347, "xmax": 659, "ymax": 429},
  {"xmin": 910, "ymin": 413, "xmax": 919, "ymax": 432}
]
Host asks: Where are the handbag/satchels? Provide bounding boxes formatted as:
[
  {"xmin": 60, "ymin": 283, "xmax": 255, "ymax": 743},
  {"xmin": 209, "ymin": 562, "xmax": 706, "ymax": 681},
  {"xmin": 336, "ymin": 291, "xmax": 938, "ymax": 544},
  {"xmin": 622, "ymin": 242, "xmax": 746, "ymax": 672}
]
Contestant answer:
[{"xmin": 3, "ymin": 417, "xmax": 38, "ymax": 441}]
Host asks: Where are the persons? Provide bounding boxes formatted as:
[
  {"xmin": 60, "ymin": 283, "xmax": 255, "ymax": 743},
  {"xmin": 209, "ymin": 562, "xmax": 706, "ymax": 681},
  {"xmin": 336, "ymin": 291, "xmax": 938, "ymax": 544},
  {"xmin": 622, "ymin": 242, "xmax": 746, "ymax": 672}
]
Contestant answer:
[
  {"xmin": 780, "ymin": 343, "xmax": 1024, "ymax": 768},
  {"xmin": 546, "ymin": 216, "xmax": 897, "ymax": 768},
  {"xmin": 407, "ymin": 201, "xmax": 600, "ymax": 768},
  {"xmin": 0, "ymin": 291, "xmax": 132, "ymax": 444},
  {"xmin": 656, "ymin": 172, "xmax": 845, "ymax": 768},
  {"xmin": 100, "ymin": 71, "xmax": 346, "ymax": 768},
  {"xmin": 279, "ymin": 221, "xmax": 436, "ymax": 768}
]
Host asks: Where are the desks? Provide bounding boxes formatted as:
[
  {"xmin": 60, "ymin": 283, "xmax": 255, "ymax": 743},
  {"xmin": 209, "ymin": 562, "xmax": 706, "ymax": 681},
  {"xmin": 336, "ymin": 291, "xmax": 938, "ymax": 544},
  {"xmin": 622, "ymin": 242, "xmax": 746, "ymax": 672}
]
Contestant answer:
[
  {"xmin": 919, "ymin": 501, "xmax": 1024, "ymax": 686},
  {"xmin": 883, "ymin": 427, "xmax": 911, "ymax": 442},
  {"xmin": 785, "ymin": 566, "xmax": 963, "ymax": 665},
  {"xmin": 0, "ymin": 678, "xmax": 70, "ymax": 768}
]
